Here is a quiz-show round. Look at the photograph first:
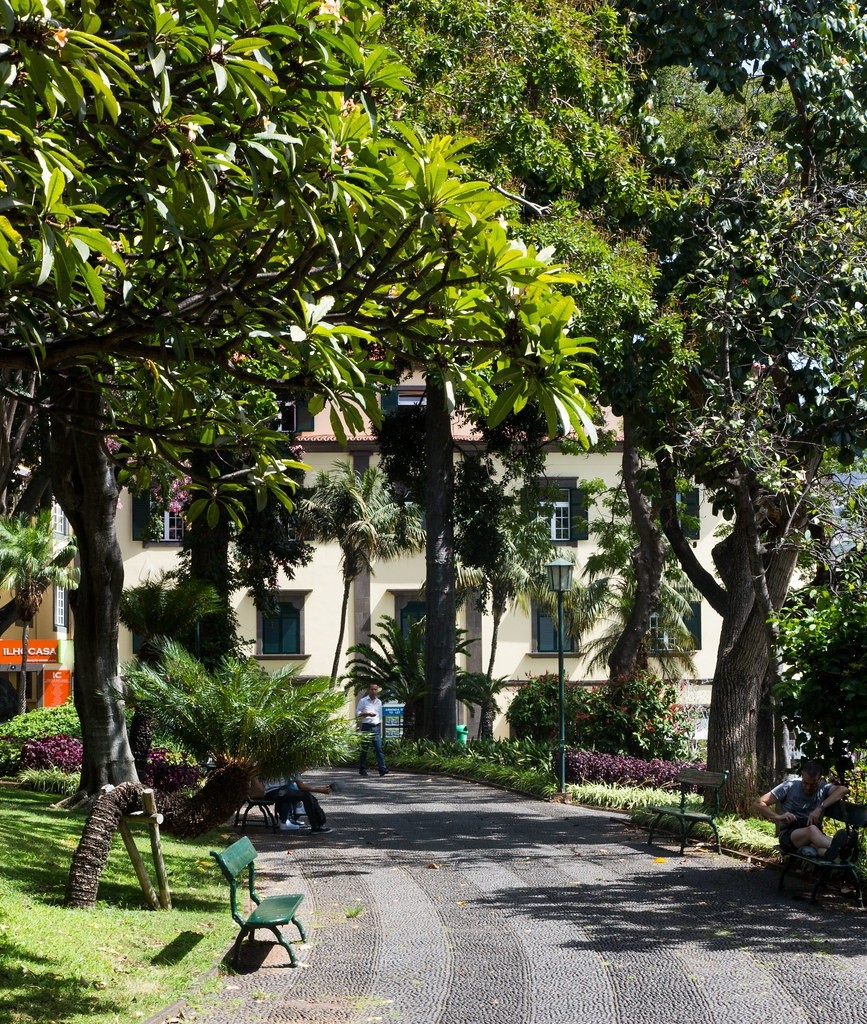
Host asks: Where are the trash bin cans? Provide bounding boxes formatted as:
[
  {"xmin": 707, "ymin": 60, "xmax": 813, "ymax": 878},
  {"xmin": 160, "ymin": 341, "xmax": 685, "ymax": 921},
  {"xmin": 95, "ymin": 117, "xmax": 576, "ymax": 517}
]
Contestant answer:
[{"xmin": 456, "ymin": 724, "xmax": 469, "ymax": 745}]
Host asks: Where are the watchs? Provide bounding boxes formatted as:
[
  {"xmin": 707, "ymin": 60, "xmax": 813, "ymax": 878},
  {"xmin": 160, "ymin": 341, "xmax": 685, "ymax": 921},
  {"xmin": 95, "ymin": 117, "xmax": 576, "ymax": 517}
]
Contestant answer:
[{"xmin": 817, "ymin": 802, "xmax": 824, "ymax": 809}]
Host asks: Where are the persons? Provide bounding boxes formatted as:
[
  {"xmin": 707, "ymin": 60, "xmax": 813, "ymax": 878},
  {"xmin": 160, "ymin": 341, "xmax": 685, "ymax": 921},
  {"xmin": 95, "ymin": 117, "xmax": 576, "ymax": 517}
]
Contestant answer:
[
  {"xmin": 250, "ymin": 776, "xmax": 333, "ymax": 834},
  {"xmin": 755, "ymin": 765, "xmax": 857, "ymax": 862},
  {"xmin": 356, "ymin": 683, "xmax": 389, "ymax": 776}
]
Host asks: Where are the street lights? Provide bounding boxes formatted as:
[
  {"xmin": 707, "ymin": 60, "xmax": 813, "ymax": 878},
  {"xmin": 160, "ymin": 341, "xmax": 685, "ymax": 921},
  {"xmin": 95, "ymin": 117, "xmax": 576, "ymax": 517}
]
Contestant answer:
[{"xmin": 545, "ymin": 557, "xmax": 575, "ymax": 800}]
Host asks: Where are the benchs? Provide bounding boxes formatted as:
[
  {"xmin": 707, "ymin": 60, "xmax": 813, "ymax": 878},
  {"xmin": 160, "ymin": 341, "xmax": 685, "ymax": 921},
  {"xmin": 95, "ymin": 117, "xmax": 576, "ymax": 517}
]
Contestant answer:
[
  {"xmin": 775, "ymin": 799, "xmax": 867, "ymax": 905},
  {"xmin": 234, "ymin": 797, "xmax": 278, "ymax": 835},
  {"xmin": 646, "ymin": 766, "xmax": 730, "ymax": 856},
  {"xmin": 208, "ymin": 835, "xmax": 308, "ymax": 966}
]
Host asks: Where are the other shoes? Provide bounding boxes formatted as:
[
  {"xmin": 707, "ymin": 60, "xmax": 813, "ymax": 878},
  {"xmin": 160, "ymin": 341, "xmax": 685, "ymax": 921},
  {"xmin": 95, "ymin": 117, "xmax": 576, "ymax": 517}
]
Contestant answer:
[
  {"xmin": 359, "ymin": 768, "xmax": 367, "ymax": 775},
  {"xmin": 824, "ymin": 829, "xmax": 859, "ymax": 861},
  {"xmin": 312, "ymin": 827, "xmax": 332, "ymax": 834},
  {"xmin": 289, "ymin": 818, "xmax": 305, "ymax": 827},
  {"xmin": 378, "ymin": 768, "xmax": 389, "ymax": 776},
  {"xmin": 280, "ymin": 819, "xmax": 299, "ymax": 829}
]
